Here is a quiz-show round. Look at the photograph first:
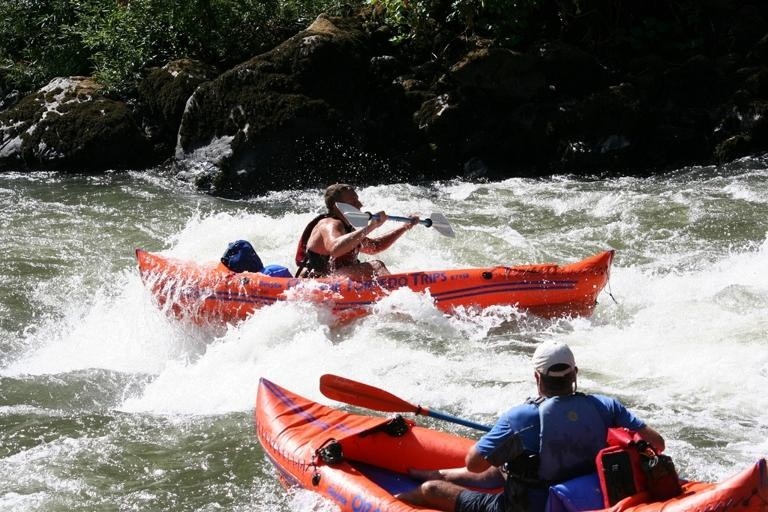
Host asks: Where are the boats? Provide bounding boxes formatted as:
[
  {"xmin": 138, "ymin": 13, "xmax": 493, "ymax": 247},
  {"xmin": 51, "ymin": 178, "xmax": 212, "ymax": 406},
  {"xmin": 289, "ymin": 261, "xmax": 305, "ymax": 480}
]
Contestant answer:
[
  {"xmin": 132, "ymin": 247, "xmax": 615, "ymax": 344},
  {"xmin": 253, "ymin": 372, "xmax": 768, "ymax": 511}
]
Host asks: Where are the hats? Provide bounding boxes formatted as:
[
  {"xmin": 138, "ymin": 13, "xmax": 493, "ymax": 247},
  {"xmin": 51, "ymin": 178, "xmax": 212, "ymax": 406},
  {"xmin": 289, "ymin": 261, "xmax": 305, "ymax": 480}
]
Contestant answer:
[{"xmin": 532, "ymin": 340, "xmax": 576, "ymax": 378}]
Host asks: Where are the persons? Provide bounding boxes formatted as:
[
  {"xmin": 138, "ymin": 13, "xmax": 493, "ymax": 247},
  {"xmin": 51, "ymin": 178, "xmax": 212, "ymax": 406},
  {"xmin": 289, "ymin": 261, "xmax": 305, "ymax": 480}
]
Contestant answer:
[
  {"xmin": 395, "ymin": 341, "xmax": 666, "ymax": 511},
  {"xmin": 296, "ymin": 183, "xmax": 419, "ymax": 276}
]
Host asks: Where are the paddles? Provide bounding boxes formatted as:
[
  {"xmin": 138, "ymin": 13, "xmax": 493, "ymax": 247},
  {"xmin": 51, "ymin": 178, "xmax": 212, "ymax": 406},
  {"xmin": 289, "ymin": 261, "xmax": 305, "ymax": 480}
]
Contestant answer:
[
  {"xmin": 335, "ymin": 201, "xmax": 455, "ymax": 238},
  {"xmin": 320, "ymin": 375, "xmax": 493, "ymax": 431}
]
